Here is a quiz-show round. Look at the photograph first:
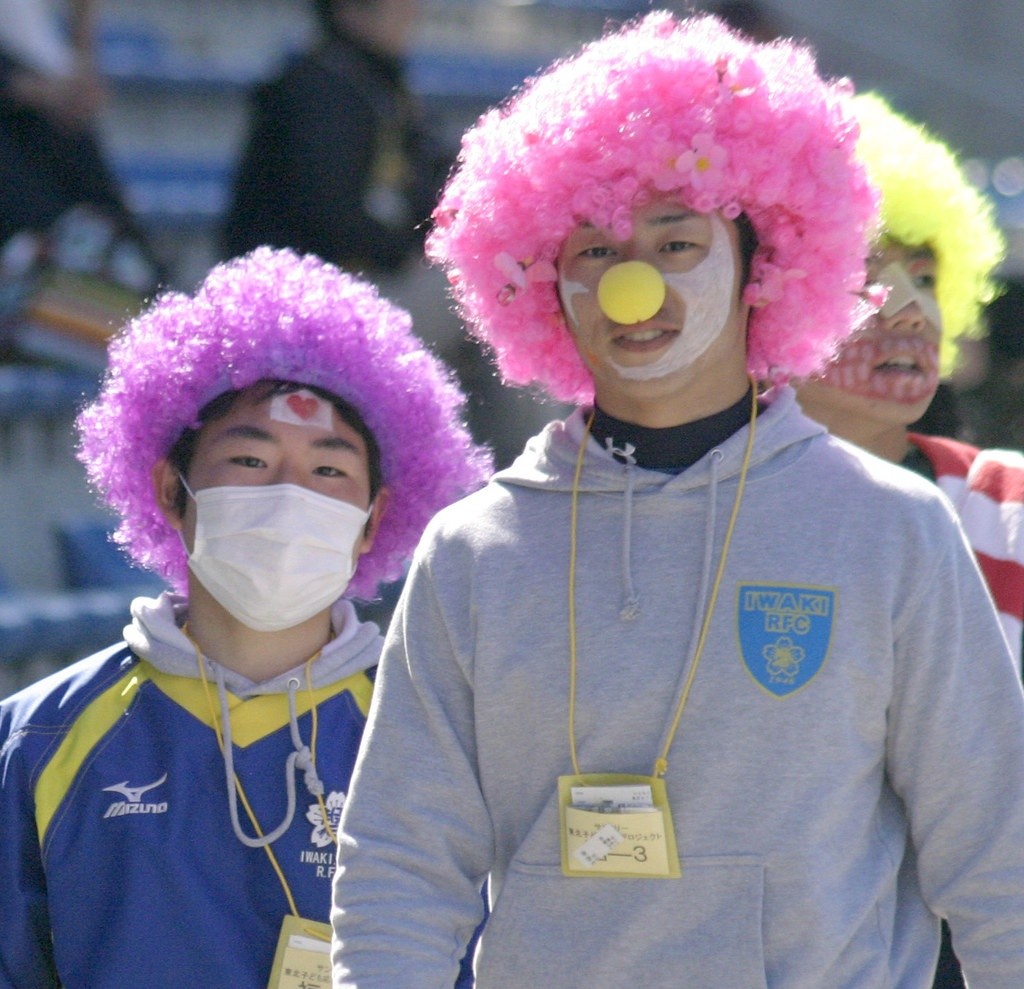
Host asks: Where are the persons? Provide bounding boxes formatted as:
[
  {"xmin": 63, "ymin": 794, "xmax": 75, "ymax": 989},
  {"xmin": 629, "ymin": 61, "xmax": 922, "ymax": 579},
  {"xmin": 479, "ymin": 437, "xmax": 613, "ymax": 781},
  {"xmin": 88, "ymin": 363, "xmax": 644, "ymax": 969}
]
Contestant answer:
[
  {"xmin": 222, "ymin": 4, "xmax": 574, "ymax": 468},
  {"xmin": 801, "ymin": 83, "xmax": 1024, "ymax": 684},
  {"xmin": 330, "ymin": 9, "xmax": 1024, "ymax": 989},
  {"xmin": 1, "ymin": 2, "xmax": 170, "ymax": 365},
  {"xmin": 0, "ymin": 247, "xmax": 492, "ymax": 989}
]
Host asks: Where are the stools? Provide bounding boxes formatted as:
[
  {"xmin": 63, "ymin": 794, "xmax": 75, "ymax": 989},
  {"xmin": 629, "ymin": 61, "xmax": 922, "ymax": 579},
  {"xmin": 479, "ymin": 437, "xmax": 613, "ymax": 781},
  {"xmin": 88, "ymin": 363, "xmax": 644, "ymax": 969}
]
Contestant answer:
[{"xmin": 1, "ymin": 0, "xmax": 651, "ymax": 667}]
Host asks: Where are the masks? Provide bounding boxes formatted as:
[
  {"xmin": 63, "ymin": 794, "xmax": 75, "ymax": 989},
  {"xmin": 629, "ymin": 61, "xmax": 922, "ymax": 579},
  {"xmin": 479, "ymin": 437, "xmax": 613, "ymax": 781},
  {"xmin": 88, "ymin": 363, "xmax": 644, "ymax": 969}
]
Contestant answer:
[{"xmin": 175, "ymin": 463, "xmax": 377, "ymax": 636}]
